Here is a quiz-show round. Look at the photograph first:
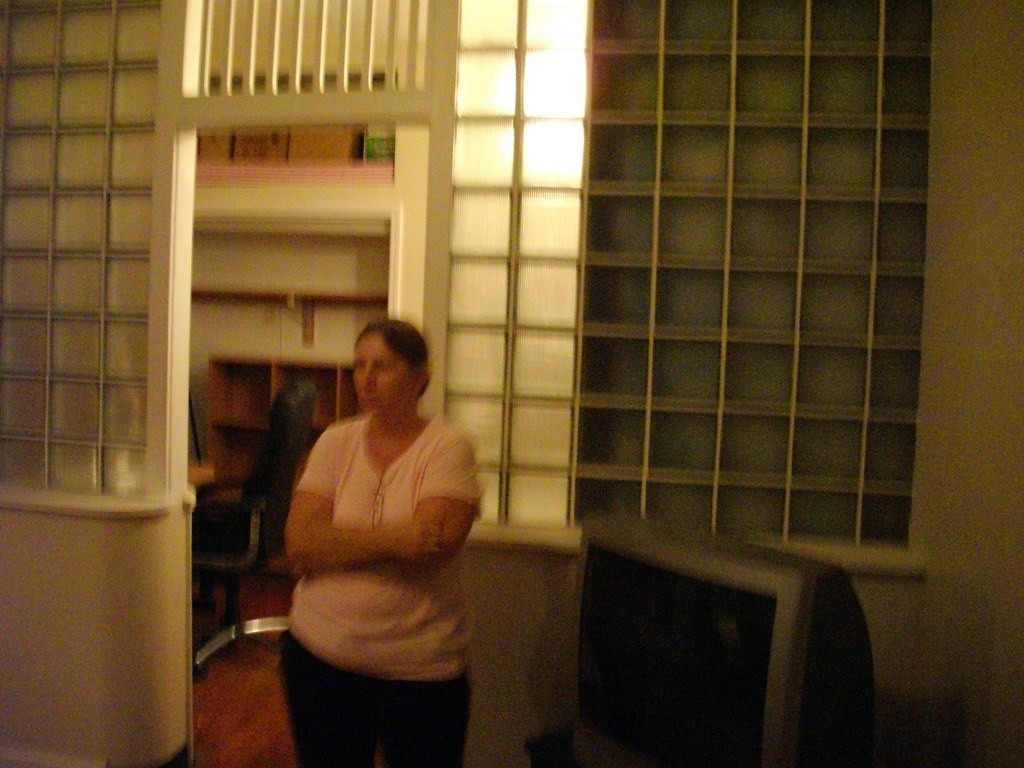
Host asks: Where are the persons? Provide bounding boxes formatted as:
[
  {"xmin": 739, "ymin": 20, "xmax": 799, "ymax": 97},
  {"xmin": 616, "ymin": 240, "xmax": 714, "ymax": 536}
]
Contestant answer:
[{"xmin": 277, "ymin": 322, "xmax": 480, "ymax": 768}]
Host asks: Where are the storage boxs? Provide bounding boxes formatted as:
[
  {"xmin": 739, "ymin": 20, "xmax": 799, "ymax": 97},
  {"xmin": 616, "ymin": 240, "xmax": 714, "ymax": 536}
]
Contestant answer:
[{"xmin": 198, "ymin": 122, "xmax": 395, "ymax": 166}]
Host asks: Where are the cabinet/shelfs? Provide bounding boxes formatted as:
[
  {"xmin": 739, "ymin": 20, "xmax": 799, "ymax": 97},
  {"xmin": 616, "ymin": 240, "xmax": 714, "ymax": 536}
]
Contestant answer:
[{"xmin": 203, "ymin": 355, "xmax": 357, "ymax": 483}]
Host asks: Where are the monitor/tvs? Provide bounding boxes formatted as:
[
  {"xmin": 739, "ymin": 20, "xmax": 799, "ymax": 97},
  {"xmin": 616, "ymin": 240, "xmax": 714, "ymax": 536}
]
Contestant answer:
[{"xmin": 572, "ymin": 510, "xmax": 876, "ymax": 767}]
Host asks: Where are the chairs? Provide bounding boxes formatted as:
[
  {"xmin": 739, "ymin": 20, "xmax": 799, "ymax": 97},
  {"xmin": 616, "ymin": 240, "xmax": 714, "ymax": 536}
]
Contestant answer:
[{"xmin": 190, "ymin": 379, "xmax": 319, "ymax": 680}]
